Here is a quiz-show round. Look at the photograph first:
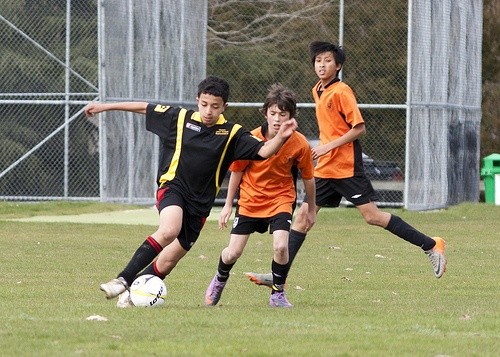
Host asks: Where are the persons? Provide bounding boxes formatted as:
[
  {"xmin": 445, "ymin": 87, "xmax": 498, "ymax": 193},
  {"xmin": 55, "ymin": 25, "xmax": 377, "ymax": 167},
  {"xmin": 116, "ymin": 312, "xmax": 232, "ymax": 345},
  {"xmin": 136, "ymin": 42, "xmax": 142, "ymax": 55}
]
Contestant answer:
[
  {"xmin": 248, "ymin": 42, "xmax": 447, "ymax": 289},
  {"xmin": 204, "ymin": 82, "xmax": 316, "ymax": 308},
  {"xmin": 84, "ymin": 77, "xmax": 298, "ymax": 308}
]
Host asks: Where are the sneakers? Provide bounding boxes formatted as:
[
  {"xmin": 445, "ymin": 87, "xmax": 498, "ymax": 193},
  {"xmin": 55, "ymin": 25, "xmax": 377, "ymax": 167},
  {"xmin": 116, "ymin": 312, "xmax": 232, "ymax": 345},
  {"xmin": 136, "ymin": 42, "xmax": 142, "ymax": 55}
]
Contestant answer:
[
  {"xmin": 100, "ymin": 277, "xmax": 129, "ymax": 299},
  {"xmin": 424, "ymin": 237, "xmax": 447, "ymax": 278},
  {"xmin": 270, "ymin": 293, "xmax": 293, "ymax": 308},
  {"xmin": 117, "ymin": 290, "xmax": 134, "ymax": 307},
  {"xmin": 205, "ymin": 275, "xmax": 226, "ymax": 306},
  {"xmin": 246, "ymin": 272, "xmax": 287, "ymax": 290}
]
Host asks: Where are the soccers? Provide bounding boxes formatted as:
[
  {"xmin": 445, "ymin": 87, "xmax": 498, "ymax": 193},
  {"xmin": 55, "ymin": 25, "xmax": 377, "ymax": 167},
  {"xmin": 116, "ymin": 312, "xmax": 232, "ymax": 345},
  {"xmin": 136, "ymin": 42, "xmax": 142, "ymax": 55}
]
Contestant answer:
[{"xmin": 130, "ymin": 274, "xmax": 167, "ymax": 307}]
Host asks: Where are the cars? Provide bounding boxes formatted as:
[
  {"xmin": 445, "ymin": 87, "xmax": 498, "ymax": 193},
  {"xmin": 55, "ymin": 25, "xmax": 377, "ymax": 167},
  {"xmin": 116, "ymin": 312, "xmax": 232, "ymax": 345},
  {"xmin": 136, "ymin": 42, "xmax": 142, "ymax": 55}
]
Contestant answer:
[{"xmin": 297, "ymin": 140, "xmax": 404, "ymax": 207}]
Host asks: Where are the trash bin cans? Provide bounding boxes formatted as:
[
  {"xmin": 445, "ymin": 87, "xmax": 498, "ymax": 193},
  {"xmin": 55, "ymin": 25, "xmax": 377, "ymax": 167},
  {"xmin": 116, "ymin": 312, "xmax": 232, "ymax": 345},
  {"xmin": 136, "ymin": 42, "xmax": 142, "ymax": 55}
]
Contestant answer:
[{"xmin": 481, "ymin": 153, "xmax": 500, "ymax": 206}]
